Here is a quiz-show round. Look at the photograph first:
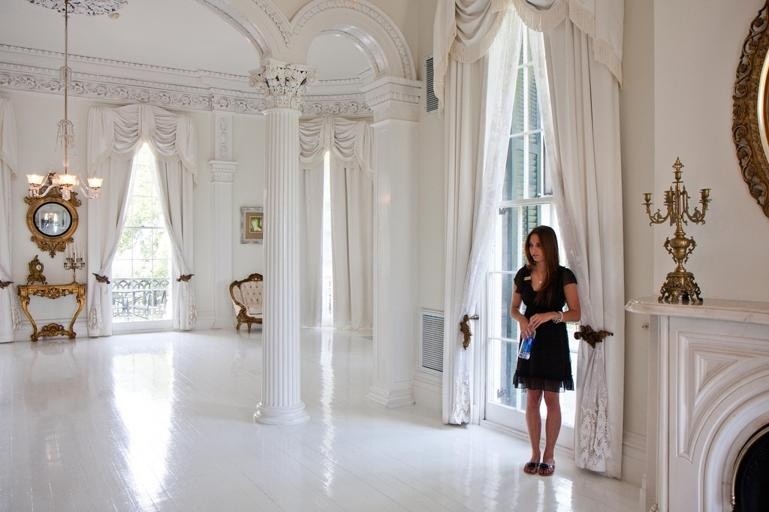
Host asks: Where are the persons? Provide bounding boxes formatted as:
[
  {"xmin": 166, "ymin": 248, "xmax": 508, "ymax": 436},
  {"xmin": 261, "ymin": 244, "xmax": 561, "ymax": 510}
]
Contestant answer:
[{"xmin": 510, "ymin": 226, "xmax": 581, "ymax": 476}]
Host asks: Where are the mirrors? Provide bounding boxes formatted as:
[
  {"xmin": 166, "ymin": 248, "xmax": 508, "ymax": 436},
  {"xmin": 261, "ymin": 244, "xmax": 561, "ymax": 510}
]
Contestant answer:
[{"xmin": 24, "ymin": 186, "xmax": 82, "ymax": 257}]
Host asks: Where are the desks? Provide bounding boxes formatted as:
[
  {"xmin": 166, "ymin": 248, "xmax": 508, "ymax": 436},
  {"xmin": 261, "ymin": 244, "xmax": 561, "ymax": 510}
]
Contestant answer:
[{"xmin": 17, "ymin": 281, "xmax": 87, "ymax": 342}]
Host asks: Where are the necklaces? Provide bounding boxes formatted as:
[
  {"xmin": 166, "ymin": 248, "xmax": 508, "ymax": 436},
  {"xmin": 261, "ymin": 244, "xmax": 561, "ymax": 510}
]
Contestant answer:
[{"xmin": 532, "ymin": 270, "xmax": 545, "ymax": 285}]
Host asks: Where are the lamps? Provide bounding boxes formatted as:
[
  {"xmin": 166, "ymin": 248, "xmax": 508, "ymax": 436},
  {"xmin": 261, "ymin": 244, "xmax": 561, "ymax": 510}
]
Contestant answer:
[{"xmin": 26, "ymin": 0, "xmax": 105, "ymax": 203}]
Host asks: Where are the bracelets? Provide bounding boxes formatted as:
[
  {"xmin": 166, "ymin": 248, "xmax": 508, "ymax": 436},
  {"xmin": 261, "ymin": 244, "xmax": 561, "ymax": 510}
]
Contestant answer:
[{"xmin": 553, "ymin": 311, "xmax": 563, "ymax": 324}]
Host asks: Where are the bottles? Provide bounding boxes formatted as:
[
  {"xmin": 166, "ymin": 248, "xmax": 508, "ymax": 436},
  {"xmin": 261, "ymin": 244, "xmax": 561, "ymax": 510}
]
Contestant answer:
[{"xmin": 516, "ymin": 336, "xmax": 533, "ymax": 359}]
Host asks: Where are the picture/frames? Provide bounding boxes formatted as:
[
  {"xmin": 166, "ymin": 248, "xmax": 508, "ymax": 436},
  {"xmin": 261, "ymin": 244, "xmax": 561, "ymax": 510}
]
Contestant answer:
[{"xmin": 238, "ymin": 206, "xmax": 262, "ymax": 245}]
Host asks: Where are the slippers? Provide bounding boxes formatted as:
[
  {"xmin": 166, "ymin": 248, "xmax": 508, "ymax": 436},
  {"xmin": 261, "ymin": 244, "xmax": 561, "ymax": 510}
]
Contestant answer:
[{"xmin": 523, "ymin": 459, "xmax": 556, "ymax": 476}]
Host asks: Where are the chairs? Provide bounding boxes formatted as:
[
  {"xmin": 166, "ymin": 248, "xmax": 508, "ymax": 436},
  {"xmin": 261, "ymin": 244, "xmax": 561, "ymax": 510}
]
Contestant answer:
[{"xmin": 229, "ymin": 273, "xmax": 264, "ymax": 333}]
